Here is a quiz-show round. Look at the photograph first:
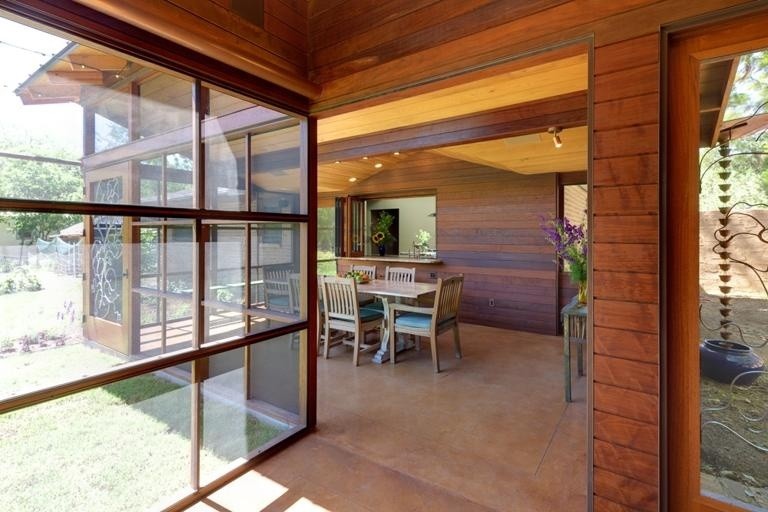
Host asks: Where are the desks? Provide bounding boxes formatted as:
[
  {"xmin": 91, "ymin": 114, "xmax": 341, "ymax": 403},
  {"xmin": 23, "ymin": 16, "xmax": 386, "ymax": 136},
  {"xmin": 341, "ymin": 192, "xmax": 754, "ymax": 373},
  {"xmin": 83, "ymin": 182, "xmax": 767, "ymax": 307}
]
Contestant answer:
[{"xmin": 561, "ymin": 295, "xmax": 586, "ymax": 404}]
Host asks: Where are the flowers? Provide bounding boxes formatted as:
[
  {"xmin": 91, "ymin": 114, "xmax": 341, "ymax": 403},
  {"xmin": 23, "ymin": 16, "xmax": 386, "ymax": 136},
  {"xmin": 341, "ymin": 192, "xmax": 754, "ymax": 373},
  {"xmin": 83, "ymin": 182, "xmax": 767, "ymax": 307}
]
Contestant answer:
[
  {"xmin": 368, "ymin": 211, "xmax": 395, "ymax": 247},
  {"xmin": 416, "ymin": 230, "xmax": 431, "ymax": 247},
  {"xmin": 540, "ymin": 214, "xmax": 588, "ymax": 281}
]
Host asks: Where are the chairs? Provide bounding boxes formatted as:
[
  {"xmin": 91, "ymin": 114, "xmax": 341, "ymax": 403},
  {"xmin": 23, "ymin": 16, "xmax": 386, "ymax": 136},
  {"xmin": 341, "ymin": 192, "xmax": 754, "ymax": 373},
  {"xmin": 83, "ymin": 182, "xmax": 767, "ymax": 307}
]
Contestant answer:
[{"xmin": 262, "ymin": 262, "xmax": 464, "ymax": 375}]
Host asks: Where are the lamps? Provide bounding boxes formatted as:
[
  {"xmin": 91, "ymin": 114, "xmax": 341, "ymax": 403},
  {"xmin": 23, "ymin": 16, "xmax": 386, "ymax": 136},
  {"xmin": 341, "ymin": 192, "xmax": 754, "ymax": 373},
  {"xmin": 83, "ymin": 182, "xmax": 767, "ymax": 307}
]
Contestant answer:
[{"xmin": 548, "ymin": 126, "xmax": 563, "ymax": 148}]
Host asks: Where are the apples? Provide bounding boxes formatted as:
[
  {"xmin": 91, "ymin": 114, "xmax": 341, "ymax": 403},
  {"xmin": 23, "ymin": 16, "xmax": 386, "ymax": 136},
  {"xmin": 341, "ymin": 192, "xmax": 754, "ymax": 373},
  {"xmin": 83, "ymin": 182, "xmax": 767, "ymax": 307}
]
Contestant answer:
[{"xmin": 337, "ymin": 271, "xmax": 368, "ymax": 281}]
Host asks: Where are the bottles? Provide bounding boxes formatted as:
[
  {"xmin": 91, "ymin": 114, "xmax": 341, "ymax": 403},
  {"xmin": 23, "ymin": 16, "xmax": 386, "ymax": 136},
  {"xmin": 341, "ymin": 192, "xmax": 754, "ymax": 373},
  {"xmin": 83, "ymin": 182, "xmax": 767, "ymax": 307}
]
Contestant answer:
[{"xmin": 415, "ymin": 246, "xmax": 420, "ymax": 257}]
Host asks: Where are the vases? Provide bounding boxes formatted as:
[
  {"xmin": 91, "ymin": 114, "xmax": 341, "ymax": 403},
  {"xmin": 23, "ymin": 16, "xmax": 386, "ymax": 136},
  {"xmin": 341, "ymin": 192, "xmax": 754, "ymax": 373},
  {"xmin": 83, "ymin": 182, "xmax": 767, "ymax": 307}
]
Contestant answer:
[
  {"xmin": 377, "ymin": 244, "xmax": 385, "ymax": 256},
  {"xmin": 696, "ymin": 341, "xmax": 764, "ymax": 384},
  {"xmin": 578, "ymin": 280, "xmax": 588, "ymax": 304}
]
